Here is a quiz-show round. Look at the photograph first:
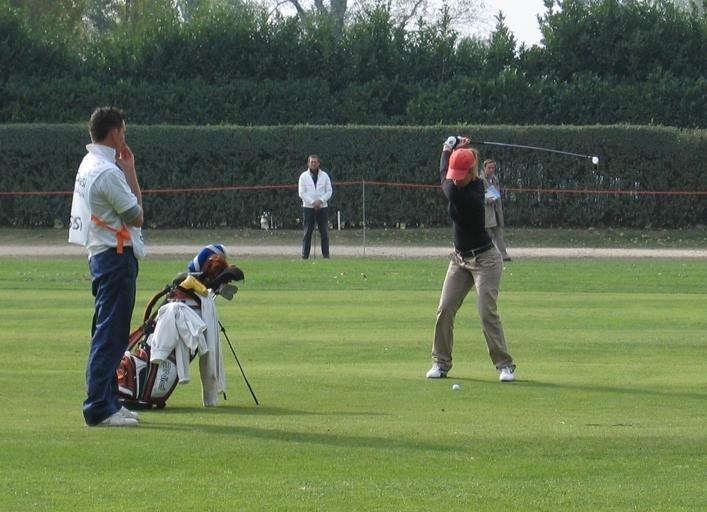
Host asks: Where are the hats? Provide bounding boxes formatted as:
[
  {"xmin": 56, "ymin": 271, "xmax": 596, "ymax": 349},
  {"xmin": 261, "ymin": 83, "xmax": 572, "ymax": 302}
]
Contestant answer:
[{"xmin": 446, "ymin": 148, "xmax": 476, "ymax": 180}]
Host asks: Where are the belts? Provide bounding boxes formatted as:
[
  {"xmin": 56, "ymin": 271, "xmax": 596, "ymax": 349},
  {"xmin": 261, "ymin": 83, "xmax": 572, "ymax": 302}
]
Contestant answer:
[{"xmin": 455, "ymin": 242, "xmax": 494, "ymax": 258}]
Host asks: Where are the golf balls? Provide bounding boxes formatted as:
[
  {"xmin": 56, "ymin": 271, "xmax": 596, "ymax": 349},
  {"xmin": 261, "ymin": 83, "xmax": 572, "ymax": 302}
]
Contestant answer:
[{"xmin": 453, "ymin": 384, "xmax": 459, "ymax": 389}]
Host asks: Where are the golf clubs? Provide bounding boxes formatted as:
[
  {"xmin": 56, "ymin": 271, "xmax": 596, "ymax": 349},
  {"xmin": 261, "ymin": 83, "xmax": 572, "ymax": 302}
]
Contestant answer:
[
  {"xmin": 313, "ymin": 211, "xmax": 317, "ymax": 259},
  {"xmin": 173, "ymin": 253, "xmax": 243, "ymax": 300},
  {"xmin": 471, "ymin": 140, "xmax": 599, "ymax": 165}
]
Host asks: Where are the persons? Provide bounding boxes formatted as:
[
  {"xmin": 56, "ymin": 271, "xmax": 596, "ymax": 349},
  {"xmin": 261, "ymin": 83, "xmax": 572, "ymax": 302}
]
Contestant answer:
[
  {"xmin": 479, "ymin": 159, "xmax": 511, "ymax": 261},
  {"xmin": 68, "ymin": 106, "xmax": 145, "ymax": 427},
  {"xmin": 298, "ymin": 154, "xmax": 332, "ymax": 259},
  {"xmin": 426, "ymin": 136, "xmax": 516, "ymax": 382}
]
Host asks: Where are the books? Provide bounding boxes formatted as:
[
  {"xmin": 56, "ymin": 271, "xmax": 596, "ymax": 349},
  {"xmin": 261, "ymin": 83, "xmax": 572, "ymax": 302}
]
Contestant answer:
[{"xmin": 485, "ymin": 185, "xmax": 501, "ymax": 200}]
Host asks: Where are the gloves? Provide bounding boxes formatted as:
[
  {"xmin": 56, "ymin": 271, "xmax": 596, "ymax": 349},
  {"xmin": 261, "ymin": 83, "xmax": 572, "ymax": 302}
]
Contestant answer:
[{"xmin": 442, "ymin": 136, "xmax": 460, "ymax": 150}]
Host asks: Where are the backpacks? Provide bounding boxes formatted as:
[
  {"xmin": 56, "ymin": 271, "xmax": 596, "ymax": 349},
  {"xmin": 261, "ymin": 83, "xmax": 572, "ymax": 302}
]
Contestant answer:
[{"xmin": 115, "ymin": 279, "xmax": 217, "ymax": 409}]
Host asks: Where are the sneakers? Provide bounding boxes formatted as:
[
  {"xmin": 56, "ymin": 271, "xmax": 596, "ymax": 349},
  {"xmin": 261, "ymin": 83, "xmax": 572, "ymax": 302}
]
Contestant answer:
[
  {"xmin": 426, "ymin": 366, "xmax": 447, "ymax": 378},
  {"xmin": 499, "ymin": 367, "xmax": 514, "ymax": 381},
  {"xmin": 97, "ymin": 405, "xmax": 138, "ymax": 426}
]
